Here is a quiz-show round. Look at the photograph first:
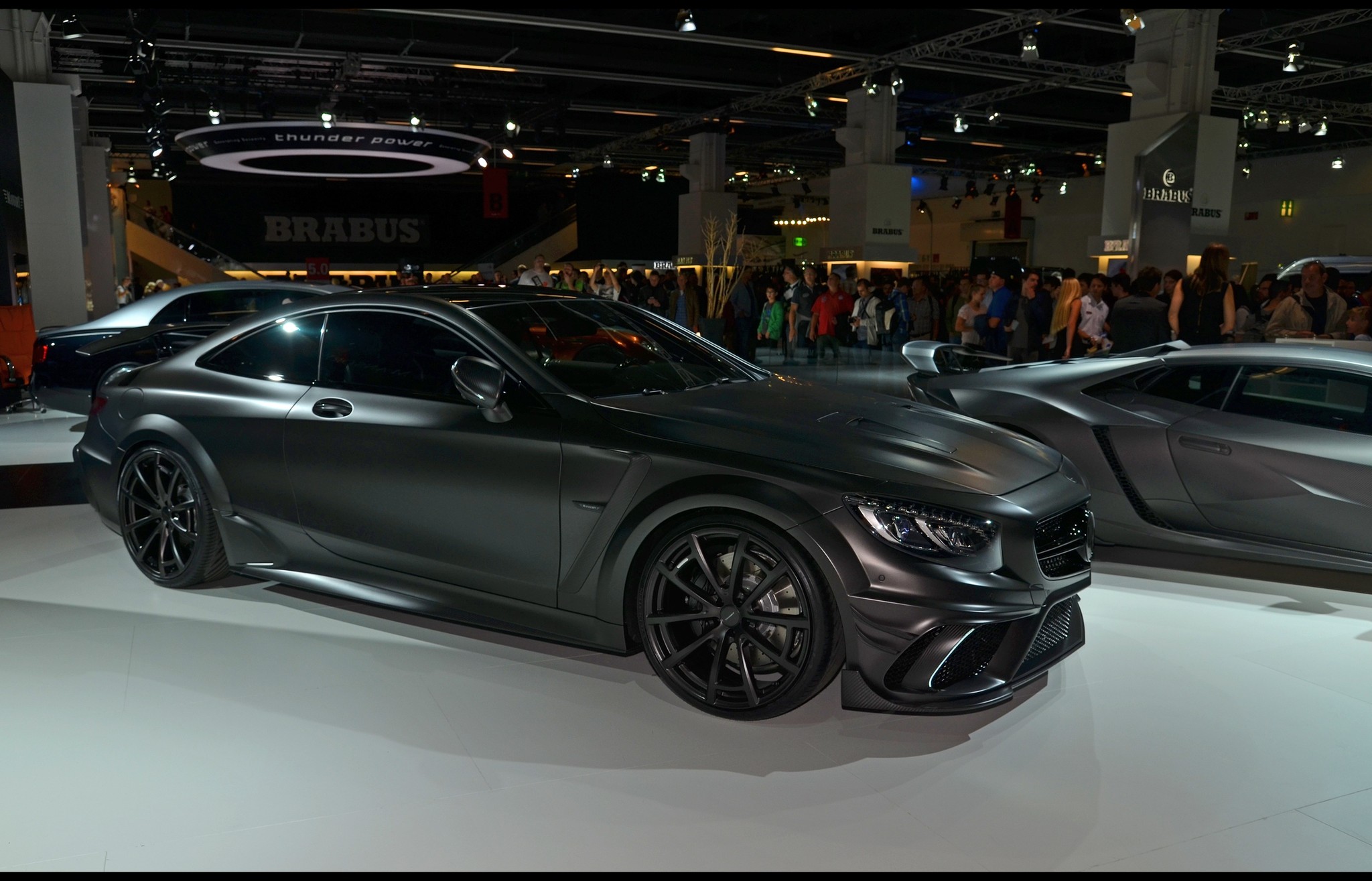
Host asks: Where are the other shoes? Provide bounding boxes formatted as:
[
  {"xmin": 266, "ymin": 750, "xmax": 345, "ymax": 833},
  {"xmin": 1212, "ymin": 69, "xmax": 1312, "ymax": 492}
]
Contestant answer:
[
  {"xmin": 755, "ymin": 357, "xmax": 762, "ymax": 364},
  {"xmin": 783, "ymin": 358, "xmax": 801, "ymax": 365}
]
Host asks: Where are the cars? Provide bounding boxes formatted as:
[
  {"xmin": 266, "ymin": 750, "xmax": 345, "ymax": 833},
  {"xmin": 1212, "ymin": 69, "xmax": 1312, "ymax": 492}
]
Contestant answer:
[
  {"xmin": 70, "ymin": 285, "xmax": 1093, "ymax": 715},
  {"xmin": 31, "ymin": 283, "xmax": 362, "ymax": 422}
]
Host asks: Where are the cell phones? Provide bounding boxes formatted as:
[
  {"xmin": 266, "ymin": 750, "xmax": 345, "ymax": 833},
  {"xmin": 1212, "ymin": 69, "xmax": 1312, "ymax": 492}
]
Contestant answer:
[
  {"xmin": 598, "ymin": 263, "xmax": 605, "ymax": 268},
  {"xmin": 847, "ymin": 315, "xmax": 857, "ymax": 323},
  {"xmin": 649, "ymin": 296, "xmax": 654, "ymax": 299}
]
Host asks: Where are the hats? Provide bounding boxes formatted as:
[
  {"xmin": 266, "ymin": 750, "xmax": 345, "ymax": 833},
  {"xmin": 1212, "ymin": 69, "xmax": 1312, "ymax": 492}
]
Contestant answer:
[{"xmin": 992, "ymin": 267, "xmax": 1011, "ymax": 283}]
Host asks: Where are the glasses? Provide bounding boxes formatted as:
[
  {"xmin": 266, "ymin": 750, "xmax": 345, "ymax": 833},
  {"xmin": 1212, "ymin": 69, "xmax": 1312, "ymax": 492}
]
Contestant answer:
[
  {"xmin": 401, "ymin": 273, "xmax": 423, "ymax": 279},
  {"xmin": 766, "ymin": 291, "xmax": 776, "ymax": 295},
  {"xmin": 1255, "ymin": 287, "xmax": 1269, "ymax": 291},
  {"xmin": 1303, "ymin": 260, "xmax": 1327, "ymax": 272}
]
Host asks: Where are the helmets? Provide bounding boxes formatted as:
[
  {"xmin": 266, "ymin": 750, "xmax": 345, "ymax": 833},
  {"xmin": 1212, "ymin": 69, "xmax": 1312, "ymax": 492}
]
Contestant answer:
[{"xmin": 396, "ymin": 257, "xmax": 425, "ymax": 273}]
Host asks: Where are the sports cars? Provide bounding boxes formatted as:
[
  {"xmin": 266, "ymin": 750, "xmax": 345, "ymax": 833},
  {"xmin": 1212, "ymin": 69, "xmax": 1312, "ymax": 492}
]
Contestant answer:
[{"xmin": 902, "ymin": 339, "xmax": 1372, "ymax": 578}]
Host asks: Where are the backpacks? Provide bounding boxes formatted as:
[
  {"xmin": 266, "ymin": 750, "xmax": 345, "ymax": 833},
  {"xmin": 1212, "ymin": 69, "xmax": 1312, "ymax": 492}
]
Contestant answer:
[{"xmin": 875, "ymin": 292, "xmax": 905, "ymax": 336}]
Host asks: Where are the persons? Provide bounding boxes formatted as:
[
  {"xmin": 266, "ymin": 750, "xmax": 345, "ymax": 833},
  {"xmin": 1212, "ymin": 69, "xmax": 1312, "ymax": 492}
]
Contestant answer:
[
  {"xmin": 227, "ymin": 254, "xmax": 1371, "ymax": 360},
  {"xmin": 1169, "ymin": 242, "xmax": 1235, "ymax": 344}
]
